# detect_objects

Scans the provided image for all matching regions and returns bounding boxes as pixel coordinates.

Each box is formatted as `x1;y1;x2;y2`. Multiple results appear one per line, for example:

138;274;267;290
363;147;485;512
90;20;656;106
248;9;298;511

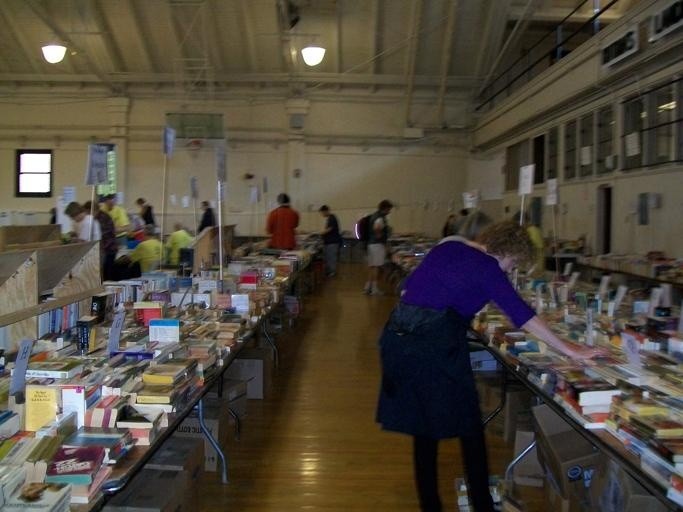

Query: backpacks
353;214;372;245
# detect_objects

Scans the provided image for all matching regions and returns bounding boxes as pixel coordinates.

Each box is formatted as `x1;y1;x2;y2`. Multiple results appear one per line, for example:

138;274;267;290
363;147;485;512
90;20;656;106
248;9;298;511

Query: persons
373;219;613;512
264;193;300;251
361;199;393;296
0;228;321;512
443;214;454;235
49;186;217;273
393;229;683;512
312;205;340;278
456;209;468;235
513;211;545;278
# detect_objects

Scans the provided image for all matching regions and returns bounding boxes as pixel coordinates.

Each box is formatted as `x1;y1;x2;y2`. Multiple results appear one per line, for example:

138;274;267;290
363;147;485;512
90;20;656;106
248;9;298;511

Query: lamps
301;36;328;67
41;33;68;64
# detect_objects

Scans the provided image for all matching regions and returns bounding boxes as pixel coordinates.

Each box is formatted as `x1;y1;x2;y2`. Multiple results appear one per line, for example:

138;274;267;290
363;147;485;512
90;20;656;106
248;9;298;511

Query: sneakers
363;287;385;297
